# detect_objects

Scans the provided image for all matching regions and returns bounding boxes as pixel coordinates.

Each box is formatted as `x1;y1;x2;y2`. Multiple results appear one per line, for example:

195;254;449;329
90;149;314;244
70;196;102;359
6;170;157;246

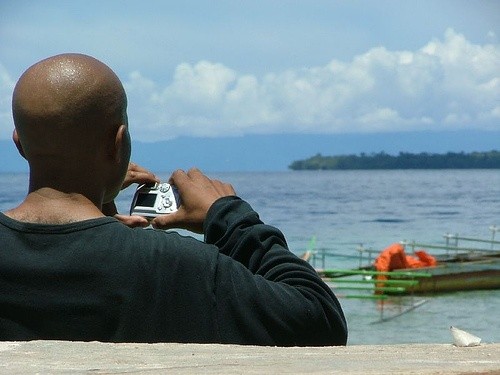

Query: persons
0;52;350;347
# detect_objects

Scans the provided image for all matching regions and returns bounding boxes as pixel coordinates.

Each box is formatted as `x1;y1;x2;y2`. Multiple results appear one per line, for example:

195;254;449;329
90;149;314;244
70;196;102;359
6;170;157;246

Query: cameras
130;182;183;225
